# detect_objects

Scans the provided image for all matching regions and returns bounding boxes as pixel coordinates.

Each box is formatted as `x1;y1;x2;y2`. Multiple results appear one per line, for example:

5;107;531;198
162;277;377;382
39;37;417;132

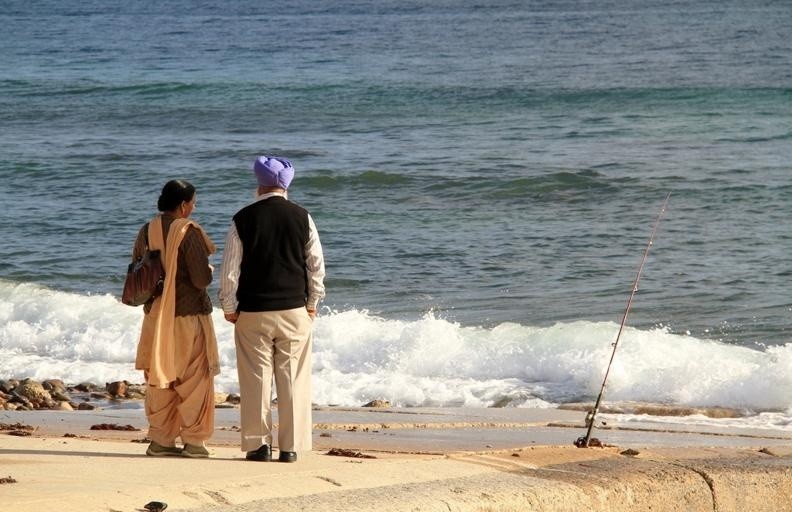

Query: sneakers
146;441;208;458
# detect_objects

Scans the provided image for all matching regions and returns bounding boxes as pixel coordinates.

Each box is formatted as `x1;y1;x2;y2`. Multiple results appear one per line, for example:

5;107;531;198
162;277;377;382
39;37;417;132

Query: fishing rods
577;191;670;447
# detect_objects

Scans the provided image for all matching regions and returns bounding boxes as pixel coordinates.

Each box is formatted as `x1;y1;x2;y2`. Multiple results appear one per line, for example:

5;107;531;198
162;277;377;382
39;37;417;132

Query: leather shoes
246;445;296;462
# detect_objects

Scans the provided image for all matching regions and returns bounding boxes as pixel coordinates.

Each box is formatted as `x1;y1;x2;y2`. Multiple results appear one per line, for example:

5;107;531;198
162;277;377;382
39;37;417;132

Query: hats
254;156;295;189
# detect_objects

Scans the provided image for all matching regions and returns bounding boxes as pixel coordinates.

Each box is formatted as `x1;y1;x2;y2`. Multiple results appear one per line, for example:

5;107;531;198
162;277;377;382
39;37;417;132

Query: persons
131;178;217;459
219;156;326;462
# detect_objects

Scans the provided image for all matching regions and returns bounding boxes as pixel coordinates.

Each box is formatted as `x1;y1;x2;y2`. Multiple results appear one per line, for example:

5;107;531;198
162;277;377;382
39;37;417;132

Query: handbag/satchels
122;250;165;306
